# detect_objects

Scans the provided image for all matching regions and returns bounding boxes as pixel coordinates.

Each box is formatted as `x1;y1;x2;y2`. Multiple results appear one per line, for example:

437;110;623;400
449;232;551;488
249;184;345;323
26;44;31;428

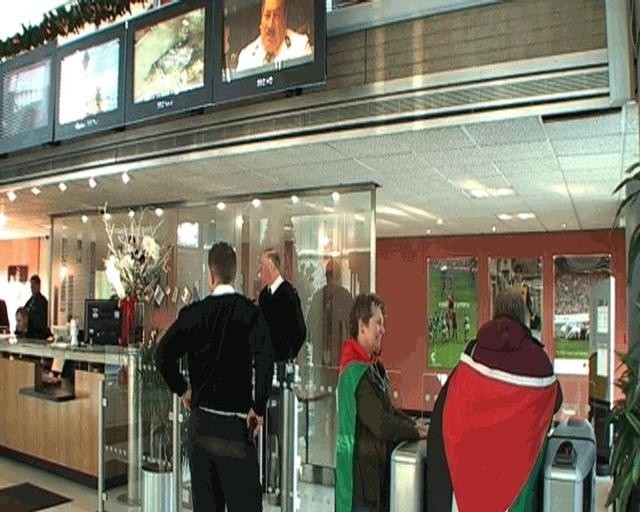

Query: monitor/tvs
85;297;118;345
212;0;328;106
54;21;124;143
0;43;55;158
126;0;211;126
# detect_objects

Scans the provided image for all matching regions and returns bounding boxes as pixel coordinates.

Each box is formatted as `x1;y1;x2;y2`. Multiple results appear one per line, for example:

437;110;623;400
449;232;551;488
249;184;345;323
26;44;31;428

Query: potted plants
126;323;189;512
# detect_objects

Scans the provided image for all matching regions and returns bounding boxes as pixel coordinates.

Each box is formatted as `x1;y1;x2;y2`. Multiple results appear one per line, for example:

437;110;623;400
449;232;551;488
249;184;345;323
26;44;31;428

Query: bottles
70;317;79;345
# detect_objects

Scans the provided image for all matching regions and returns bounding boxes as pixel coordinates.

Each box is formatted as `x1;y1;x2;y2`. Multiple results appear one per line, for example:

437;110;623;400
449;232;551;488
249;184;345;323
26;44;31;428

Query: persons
427;259;476;344
257;247;307;360
496;257;536;328
426;286;562;512
307;262;354;461
335;292;425;512
555;272;609;341
24;276;47;338
237;1;311;71
156;242;273;511
16;308;37;338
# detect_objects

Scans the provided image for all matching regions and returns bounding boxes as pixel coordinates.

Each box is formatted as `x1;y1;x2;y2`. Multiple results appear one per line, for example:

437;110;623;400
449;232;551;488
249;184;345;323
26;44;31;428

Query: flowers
97;201;172;303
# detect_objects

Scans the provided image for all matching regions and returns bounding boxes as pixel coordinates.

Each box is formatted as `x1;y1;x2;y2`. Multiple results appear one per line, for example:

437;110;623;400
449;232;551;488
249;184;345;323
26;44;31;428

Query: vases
120;297;137;348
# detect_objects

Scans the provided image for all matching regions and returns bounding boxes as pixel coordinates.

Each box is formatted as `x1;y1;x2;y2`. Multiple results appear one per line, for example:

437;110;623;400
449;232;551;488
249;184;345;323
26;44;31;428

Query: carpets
0;482;72;512
